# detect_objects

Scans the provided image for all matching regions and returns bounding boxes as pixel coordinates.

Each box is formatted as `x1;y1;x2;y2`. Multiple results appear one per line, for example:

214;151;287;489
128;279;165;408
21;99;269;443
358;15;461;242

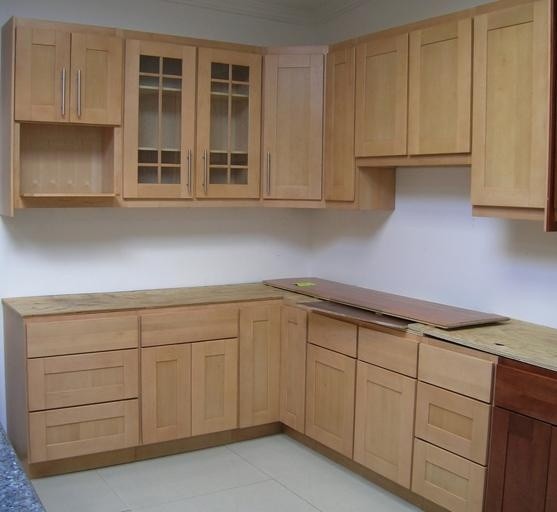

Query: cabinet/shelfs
239;298;282;440
411;335;499;512
3;304;140;479
262;45;325;210
0;17;124;219
483;355;556;511
324;40;395;212
473;1;557;231
124;29;261;208
355;9;472;167
282;304;307;445
308;308;419;507
140;302;239;463
